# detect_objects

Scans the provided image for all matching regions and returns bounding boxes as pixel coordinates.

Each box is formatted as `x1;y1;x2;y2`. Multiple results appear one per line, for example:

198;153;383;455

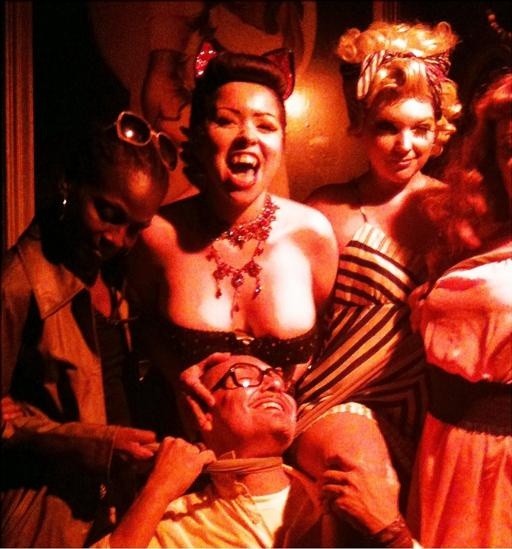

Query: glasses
209;362;289;390
103;111;177;172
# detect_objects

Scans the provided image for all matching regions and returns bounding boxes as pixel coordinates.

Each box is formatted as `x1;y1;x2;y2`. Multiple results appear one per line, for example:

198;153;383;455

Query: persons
1;23;511;547
